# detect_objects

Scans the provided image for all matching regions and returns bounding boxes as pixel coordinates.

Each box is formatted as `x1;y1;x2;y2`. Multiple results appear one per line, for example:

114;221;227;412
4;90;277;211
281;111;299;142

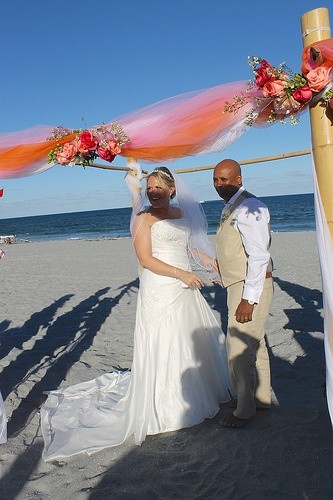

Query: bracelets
174;267;177;279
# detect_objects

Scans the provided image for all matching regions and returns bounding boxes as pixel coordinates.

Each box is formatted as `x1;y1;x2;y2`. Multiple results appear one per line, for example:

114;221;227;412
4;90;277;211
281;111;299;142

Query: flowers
222;55;333;127
46;123;131;168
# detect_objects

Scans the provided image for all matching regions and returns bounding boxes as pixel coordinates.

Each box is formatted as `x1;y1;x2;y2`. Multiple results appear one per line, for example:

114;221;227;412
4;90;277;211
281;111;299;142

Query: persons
214;159;274;426
129;167;237;451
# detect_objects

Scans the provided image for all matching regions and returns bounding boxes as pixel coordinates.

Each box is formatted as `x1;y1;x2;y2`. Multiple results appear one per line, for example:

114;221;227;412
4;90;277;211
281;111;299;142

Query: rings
245;317;248;319
